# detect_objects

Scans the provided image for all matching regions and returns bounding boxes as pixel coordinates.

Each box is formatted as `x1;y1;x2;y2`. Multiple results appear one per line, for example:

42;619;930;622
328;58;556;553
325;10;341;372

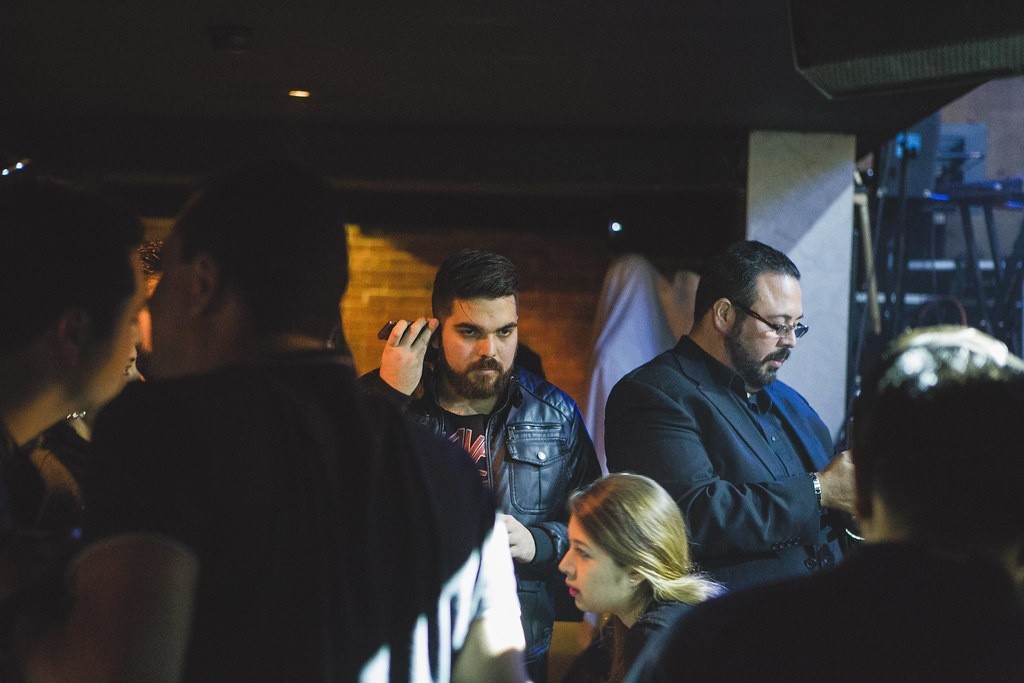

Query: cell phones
378;321;441;343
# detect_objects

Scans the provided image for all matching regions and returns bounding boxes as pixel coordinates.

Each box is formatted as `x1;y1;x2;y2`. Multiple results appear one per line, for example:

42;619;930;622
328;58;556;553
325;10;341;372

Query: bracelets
810;471;825;511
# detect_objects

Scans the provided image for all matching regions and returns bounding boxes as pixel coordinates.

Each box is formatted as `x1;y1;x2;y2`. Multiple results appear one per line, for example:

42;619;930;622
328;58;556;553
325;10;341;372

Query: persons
3;178;1023;683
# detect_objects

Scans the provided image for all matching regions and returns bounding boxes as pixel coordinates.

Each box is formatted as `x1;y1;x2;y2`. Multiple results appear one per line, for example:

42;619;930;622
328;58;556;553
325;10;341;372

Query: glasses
737;307;809;339
